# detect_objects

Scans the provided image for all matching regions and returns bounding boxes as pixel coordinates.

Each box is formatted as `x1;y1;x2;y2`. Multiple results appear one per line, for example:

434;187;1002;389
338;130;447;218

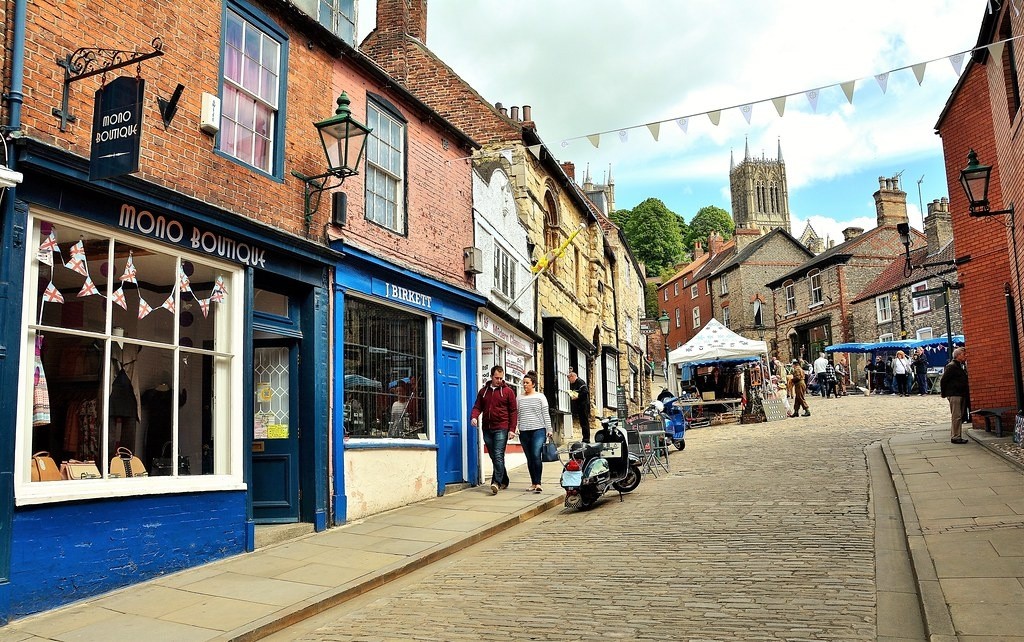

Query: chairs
622;412;670;479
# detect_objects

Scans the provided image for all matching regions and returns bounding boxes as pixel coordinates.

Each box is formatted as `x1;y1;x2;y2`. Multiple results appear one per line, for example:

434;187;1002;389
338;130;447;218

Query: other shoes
535;485;543;493
891;393;898;396
501;484;506;489
790;413;799;417
490;482;499;493
880;392;884;394
801;412;811;416
871;391;876;394
526;486;535;491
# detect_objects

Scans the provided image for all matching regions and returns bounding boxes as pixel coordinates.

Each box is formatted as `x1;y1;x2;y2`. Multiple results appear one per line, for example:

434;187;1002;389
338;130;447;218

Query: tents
669;318;774;399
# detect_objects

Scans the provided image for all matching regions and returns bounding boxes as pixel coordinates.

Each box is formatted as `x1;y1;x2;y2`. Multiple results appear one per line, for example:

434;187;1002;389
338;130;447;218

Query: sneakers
951;437;968;444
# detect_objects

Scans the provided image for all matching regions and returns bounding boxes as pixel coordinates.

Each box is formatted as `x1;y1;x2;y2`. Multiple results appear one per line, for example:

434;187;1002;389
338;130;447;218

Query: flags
530;228;582;272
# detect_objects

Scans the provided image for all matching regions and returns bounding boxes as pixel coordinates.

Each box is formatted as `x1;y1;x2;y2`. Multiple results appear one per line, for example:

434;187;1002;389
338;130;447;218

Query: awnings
825;335;965;367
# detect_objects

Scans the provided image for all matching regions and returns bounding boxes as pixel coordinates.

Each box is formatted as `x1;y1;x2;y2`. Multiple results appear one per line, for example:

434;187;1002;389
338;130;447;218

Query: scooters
553;415;643;512
642;388;687;456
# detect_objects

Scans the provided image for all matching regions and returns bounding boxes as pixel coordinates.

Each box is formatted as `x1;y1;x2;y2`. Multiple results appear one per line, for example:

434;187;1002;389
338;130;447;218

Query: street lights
658;308;670;387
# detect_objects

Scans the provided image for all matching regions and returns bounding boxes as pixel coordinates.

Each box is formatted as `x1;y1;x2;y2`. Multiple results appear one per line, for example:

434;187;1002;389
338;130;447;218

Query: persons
142;384;187;470
804;353;849;398
865;346;928;396
392;375;415;420
516;370;553;492
770;357;777;375
788;360;811;418
709;368;725;397
661;358;667;381
471;365;517;495
94;327;142;468
650;357;654;381
940;348;969;444
569;372;590;444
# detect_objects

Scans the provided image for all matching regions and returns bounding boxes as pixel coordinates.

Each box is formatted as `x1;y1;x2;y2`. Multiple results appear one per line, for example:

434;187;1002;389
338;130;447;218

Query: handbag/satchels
58;459;101;480
150;441;191;476
31;451;62;482
905;371;910;377
541;435;560;462
110;447;148;478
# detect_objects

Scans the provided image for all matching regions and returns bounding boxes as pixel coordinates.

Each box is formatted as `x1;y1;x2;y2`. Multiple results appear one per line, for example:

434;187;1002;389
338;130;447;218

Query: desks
639;430;669;476
680;398;743;429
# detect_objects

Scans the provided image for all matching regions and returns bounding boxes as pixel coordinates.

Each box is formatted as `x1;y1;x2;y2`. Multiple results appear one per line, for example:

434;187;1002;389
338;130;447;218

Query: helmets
650;400;664;412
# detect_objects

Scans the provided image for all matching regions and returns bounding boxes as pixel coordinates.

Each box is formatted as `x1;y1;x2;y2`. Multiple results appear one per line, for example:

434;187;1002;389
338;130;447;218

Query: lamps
290;90;373;226
958;147;1015;227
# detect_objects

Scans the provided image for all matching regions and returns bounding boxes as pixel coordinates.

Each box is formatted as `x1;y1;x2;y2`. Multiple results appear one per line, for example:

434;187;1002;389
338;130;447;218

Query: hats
791;359;799;363
